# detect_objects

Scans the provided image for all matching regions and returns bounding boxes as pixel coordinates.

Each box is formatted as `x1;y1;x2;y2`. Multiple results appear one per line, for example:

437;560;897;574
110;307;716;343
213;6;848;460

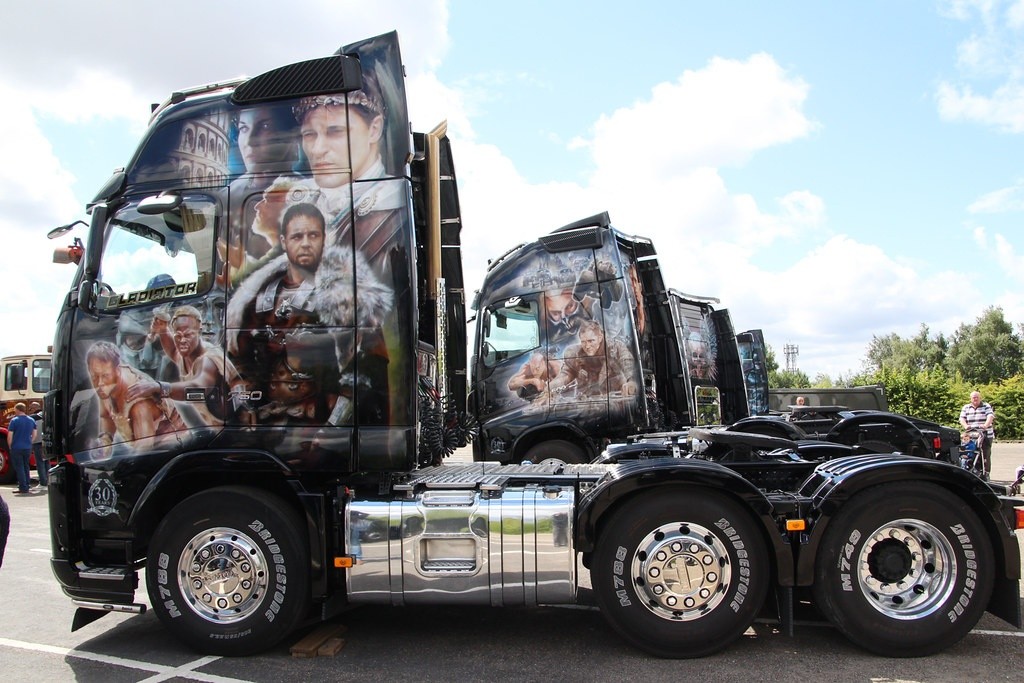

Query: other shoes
12;490;21;493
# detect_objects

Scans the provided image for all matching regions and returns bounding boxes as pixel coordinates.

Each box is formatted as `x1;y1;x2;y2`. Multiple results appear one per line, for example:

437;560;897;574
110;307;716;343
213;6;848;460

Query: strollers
958;427;990;482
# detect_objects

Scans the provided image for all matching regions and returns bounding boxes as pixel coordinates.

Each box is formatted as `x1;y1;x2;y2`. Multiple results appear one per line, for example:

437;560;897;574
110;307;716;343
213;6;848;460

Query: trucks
0;345;53;487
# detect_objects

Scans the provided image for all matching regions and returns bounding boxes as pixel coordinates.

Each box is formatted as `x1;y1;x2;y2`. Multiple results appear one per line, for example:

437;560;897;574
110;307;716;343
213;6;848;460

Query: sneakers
33;483;48;491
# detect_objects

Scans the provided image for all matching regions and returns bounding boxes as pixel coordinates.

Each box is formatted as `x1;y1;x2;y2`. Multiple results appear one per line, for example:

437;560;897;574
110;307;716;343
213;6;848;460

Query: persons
958;391;995;473
796;397;805;406
7;403;37;494
87;70;410;462
29;402;51;490
509;253;654;417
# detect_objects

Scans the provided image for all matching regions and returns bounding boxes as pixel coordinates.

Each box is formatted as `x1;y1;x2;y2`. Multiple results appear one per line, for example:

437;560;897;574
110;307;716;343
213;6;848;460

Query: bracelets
99;432;113;442
158;381;169;398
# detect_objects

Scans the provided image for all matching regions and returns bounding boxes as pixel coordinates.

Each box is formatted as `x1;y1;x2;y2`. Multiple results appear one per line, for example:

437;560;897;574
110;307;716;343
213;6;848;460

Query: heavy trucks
31;28;1024;661
464;208;963;466
